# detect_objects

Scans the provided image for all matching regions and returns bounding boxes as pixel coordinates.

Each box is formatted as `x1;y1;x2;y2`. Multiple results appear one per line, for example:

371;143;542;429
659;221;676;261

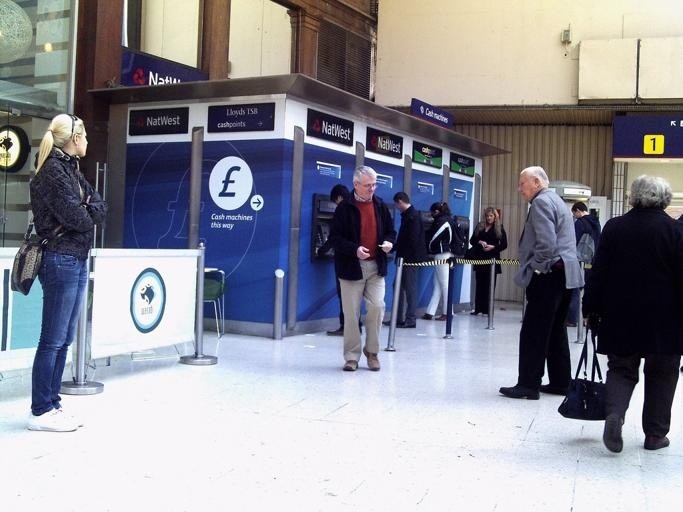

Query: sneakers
363;347;381;372
344;359;358;370
26;407;86;432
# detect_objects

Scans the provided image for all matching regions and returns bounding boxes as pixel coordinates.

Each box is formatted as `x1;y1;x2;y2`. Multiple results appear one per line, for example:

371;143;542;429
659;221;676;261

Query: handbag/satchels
465;246;476;260
557;378;609;422
11;241;43;295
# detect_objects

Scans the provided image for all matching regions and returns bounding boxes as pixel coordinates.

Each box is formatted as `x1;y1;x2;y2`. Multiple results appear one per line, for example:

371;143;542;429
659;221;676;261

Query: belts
361;255;376;262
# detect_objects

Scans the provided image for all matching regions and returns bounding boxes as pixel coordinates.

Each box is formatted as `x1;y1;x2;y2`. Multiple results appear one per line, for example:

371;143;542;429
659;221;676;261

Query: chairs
196;267;226;339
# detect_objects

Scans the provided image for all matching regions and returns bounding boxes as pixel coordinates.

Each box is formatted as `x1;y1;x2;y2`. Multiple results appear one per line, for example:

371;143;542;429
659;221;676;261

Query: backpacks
574;233;594;267
449;219;468;256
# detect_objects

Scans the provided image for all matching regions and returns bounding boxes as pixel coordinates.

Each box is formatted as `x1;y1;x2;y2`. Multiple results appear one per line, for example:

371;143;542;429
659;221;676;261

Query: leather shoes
435;314;453;321
397;318;417;328
541;380;570;395
603;411;624;453
383;319;391;326
326;326;346;336
499;383;541;402
643;434;670;450
421;313;432;320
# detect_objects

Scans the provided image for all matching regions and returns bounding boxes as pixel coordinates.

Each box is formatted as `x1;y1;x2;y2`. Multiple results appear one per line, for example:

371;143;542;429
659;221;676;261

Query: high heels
470;309;484;316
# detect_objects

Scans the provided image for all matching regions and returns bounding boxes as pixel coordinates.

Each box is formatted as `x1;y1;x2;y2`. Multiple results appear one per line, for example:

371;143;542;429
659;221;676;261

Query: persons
565;201;602;327
582;174;683;453
25;113;109;432
498;166;586;400
333;165;396;371
419;201;456;321
314;184;363;337
382;191;427;329
469;206;508;317
